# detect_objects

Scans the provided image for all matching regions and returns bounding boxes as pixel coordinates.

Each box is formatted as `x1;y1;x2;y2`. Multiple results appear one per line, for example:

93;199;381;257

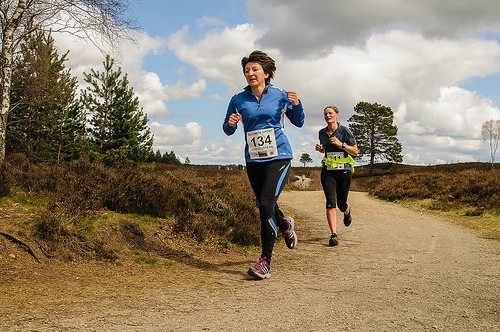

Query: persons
223;50;305;279
316;106;358;246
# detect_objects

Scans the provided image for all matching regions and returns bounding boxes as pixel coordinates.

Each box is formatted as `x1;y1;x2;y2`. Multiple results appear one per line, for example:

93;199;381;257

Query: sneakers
247;257;272;280
280;217;297;248
343;212;352;227
329;234;338;245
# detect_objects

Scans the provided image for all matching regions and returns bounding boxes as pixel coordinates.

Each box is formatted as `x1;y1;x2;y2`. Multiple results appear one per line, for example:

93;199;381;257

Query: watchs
339;142;346;149
